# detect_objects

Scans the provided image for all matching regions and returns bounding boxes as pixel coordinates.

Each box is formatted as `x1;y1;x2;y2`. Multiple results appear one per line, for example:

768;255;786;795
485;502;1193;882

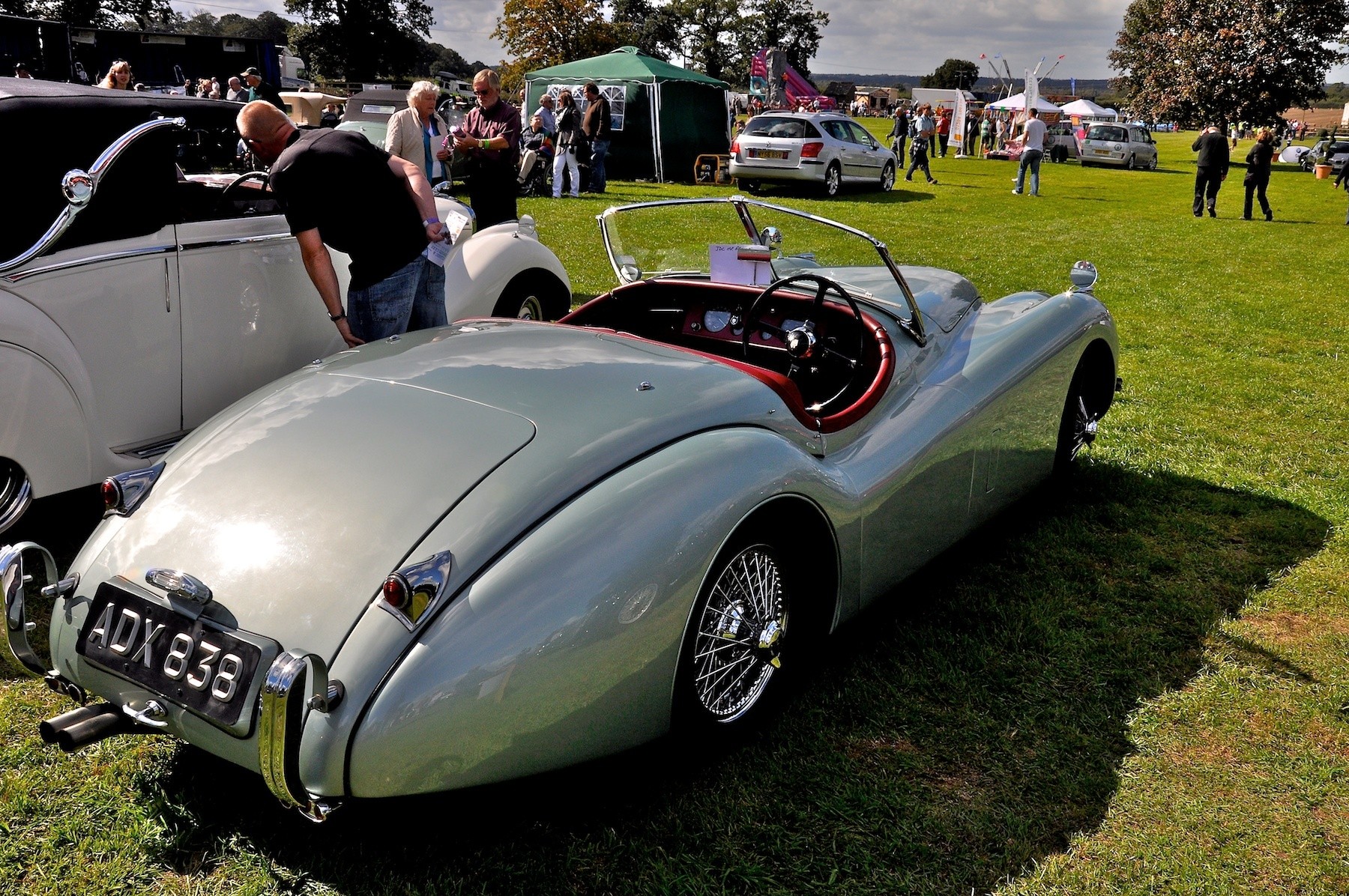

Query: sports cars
0;194;1123;832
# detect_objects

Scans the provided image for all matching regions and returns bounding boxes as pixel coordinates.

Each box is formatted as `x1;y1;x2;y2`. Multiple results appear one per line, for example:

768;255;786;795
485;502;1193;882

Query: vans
1043;120;1158;171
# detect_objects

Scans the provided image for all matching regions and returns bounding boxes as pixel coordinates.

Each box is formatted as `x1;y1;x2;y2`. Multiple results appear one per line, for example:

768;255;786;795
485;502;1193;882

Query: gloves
568;144;575;154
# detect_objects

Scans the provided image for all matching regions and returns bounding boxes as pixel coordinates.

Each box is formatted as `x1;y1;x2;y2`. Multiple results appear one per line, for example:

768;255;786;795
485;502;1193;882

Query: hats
1206;123;1220;129
239;67;261;77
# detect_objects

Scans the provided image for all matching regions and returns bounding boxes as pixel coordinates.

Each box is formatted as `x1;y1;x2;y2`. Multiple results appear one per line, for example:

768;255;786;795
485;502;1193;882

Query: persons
884;102;980;171
1238;130;1274;222
320;102;344;128
1172;119;1308;152
850;100;880;118
903;103;938;185
977;115;1007;158
746;95;821;117
91;59;135;91
520;82;612;198
236;100;451;350
382;80;457;191
454;67;522;234
135;67;288;116
1011;108;1049;196
1191;124;1230;218
1333;155;1349;226
12;61;36;79
728;93;746;135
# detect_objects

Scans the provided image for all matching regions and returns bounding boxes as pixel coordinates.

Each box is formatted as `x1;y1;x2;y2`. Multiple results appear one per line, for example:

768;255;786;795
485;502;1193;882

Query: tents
523;44;732;182
1058;98;1116;124
983;91;1064;145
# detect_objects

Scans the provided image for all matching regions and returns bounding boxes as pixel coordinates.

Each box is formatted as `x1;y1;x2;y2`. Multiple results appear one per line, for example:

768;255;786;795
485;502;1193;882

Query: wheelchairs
515;131;570;196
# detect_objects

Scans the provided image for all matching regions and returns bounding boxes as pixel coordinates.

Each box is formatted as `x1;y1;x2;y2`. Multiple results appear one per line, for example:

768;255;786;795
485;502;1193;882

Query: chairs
1041;147;1059;164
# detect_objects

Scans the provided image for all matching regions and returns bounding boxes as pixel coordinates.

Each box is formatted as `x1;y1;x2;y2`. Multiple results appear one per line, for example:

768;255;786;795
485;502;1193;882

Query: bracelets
484;138;489;149
327;306;345;322
423;217;439;227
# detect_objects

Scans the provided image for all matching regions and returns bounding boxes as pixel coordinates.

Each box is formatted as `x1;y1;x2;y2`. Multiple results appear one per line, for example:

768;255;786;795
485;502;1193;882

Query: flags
1041;56;1045;61
980;54;987;59
1059;55;1066;59
995;51;1002;58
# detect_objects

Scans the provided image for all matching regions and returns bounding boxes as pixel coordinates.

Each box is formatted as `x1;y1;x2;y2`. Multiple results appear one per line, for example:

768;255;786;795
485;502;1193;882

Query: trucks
910;87;979;111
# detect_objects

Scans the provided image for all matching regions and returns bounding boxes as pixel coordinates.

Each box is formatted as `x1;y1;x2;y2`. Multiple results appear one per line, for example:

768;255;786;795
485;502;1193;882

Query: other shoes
515;176;525;186
584;190;595;195
1207;206;1217;218
1195;214;1202;218
1239;216;1252;221
1264;208;1273;222
1027;193;1032;197
1011;189;1020;196
937;155;943;158
903;178;913;183
1011;178;1019;182
930;178;938;185
551;195;558;199
567;195;575;199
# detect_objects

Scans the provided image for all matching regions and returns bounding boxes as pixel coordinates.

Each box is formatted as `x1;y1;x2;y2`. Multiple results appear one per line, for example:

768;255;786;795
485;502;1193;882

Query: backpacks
908;118;918;137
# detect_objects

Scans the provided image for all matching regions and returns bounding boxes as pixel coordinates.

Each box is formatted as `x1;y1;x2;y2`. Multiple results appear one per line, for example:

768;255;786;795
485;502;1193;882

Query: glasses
472;87;490;96
547;100;554;103
558;99;562;103
245;137;262;157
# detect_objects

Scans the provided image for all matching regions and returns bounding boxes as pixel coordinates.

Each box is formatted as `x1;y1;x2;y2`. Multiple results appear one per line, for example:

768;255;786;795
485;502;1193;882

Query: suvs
728;109;898;197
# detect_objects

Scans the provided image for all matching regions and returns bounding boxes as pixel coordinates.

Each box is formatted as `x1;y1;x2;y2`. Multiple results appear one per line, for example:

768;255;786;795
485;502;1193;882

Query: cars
1300;141;1349;173
1;75;571;564
278;89;456;181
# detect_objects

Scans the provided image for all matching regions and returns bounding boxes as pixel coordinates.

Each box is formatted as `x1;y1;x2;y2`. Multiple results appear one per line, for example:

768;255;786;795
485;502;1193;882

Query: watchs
476;138;483;148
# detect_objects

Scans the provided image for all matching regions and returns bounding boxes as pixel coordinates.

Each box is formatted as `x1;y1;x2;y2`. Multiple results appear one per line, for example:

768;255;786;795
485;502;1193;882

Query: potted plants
1269;126;1284;162
1306;125;1337;178
